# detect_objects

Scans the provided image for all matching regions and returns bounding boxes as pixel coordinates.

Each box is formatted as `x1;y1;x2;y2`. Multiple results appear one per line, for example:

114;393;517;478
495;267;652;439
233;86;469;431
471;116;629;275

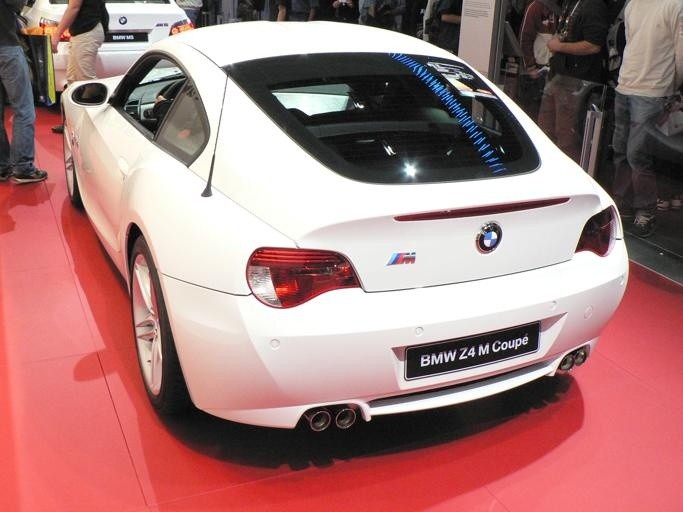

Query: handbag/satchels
18;33;56;107
533;32;555;65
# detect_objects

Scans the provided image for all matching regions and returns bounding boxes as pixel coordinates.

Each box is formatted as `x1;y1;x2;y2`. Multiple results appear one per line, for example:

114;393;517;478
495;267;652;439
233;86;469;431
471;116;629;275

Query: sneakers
0;169;14;180
656;196;682;211
620;211;659;237
15;169;47;183
51;125;64;132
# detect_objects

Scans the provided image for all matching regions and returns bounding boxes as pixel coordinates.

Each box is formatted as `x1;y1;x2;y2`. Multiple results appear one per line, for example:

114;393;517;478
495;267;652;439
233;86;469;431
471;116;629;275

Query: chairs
373;74;458;159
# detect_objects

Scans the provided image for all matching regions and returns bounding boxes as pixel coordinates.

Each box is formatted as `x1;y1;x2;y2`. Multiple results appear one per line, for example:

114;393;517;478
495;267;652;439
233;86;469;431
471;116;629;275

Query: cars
14;0;195;114
60;20;630;432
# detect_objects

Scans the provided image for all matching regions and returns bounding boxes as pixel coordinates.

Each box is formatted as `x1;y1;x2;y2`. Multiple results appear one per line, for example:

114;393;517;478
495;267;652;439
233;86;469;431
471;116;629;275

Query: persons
194;0;463;57
51;0;105;134
150;94;202;136
498;1;626;163
0;0;48;184
609;0;681;239
